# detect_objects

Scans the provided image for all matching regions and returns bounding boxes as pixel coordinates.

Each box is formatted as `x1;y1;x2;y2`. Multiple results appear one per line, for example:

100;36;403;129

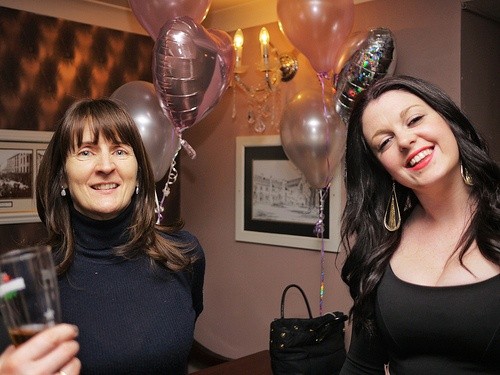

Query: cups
0;244;64;348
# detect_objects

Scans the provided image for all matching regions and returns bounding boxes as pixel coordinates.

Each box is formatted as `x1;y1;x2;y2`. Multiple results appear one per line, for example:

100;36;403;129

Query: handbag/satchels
270;284;349;375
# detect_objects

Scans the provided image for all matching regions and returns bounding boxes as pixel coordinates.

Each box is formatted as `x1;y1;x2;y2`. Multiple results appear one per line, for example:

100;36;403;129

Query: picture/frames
235;135;341;254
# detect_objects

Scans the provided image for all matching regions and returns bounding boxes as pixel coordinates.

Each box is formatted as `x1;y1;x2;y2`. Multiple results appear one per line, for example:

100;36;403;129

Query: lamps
229;27;297;134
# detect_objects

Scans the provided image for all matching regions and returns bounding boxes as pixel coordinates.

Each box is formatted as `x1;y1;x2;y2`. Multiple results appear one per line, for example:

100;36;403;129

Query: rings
58;370;69;375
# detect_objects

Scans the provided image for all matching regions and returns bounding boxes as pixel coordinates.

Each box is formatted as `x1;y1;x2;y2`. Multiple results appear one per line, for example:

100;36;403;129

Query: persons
0;99;206;375
339;75;500;375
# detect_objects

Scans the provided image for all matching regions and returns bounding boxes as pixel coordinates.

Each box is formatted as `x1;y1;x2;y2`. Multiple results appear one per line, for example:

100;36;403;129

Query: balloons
153;16;236;133
129;0;212;42
109;81;177;182
277;0;354;73
279;90;347;190
334;26;398;125
